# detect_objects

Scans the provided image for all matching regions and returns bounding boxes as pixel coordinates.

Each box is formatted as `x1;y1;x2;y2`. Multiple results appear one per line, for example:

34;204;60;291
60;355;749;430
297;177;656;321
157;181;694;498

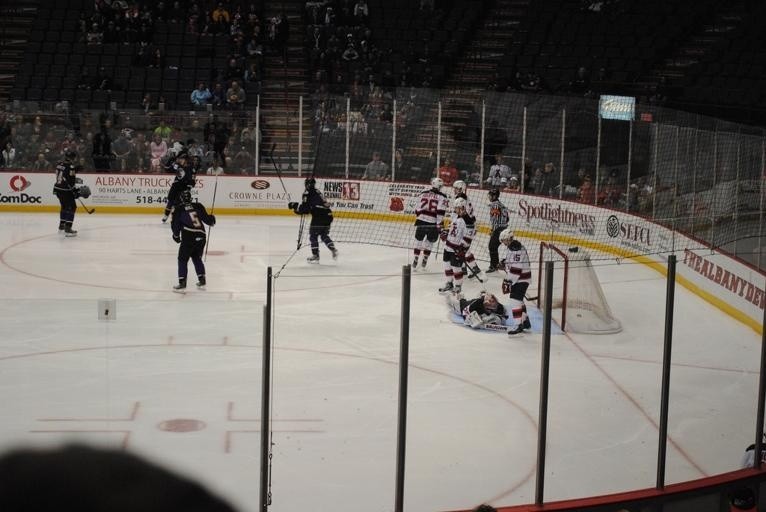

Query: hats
509;176;517;180
500;177;507;183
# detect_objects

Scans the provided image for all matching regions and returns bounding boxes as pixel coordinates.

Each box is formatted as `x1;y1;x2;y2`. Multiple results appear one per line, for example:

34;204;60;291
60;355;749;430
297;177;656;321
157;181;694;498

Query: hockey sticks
270;144;291;203
204;174;217;262
452;245;489;283
62;176;95;214
503;268;539;301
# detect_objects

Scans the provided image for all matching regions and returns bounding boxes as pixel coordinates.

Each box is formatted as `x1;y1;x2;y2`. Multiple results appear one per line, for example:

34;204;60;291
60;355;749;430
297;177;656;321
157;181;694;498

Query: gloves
436;224;442;231
288;202;298;209
172;234;180;242
501;279;511;294
440;230;449;241
73;192;80;198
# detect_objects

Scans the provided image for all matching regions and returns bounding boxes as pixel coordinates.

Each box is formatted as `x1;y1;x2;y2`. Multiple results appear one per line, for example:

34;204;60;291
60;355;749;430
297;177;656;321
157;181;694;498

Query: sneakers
59;225;65;229
64;226;77;233
307;255;318;260
412;256;418;267
523;317;530;329
468;268;480;278
485;267;496;273
422;256;428;267
173;281;186;289
162;215;167;222
439;282;453;292
196;277;206;286
332;248;337;257
508;325;524;334
452;285;461;292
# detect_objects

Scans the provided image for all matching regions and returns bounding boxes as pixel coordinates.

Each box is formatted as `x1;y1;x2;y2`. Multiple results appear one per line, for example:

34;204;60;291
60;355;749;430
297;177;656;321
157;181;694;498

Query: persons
411;177;450;272
440;154;481;188
416;152;437;183
52;152;89;236
161;151;196;222
440;180;483;280
393;149;412;182
498;228;532;337
521;158;708;221
171;191;216;295
1;0;299;173
299;1;659;139
440;291;509;328
361;152;392;184
481;153;521;193
485;188;510;274
295;177;339;264
438;197;476;302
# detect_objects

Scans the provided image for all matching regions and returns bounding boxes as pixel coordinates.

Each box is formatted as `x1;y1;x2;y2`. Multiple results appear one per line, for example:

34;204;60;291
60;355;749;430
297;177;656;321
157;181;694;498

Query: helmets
178;154;188;158
65;151;75;158
498;229;513;242
431;178;443;188
453;180;466;190
305;177;316;184
454;197;466;207
179;191;191;202
487;189;499;199
